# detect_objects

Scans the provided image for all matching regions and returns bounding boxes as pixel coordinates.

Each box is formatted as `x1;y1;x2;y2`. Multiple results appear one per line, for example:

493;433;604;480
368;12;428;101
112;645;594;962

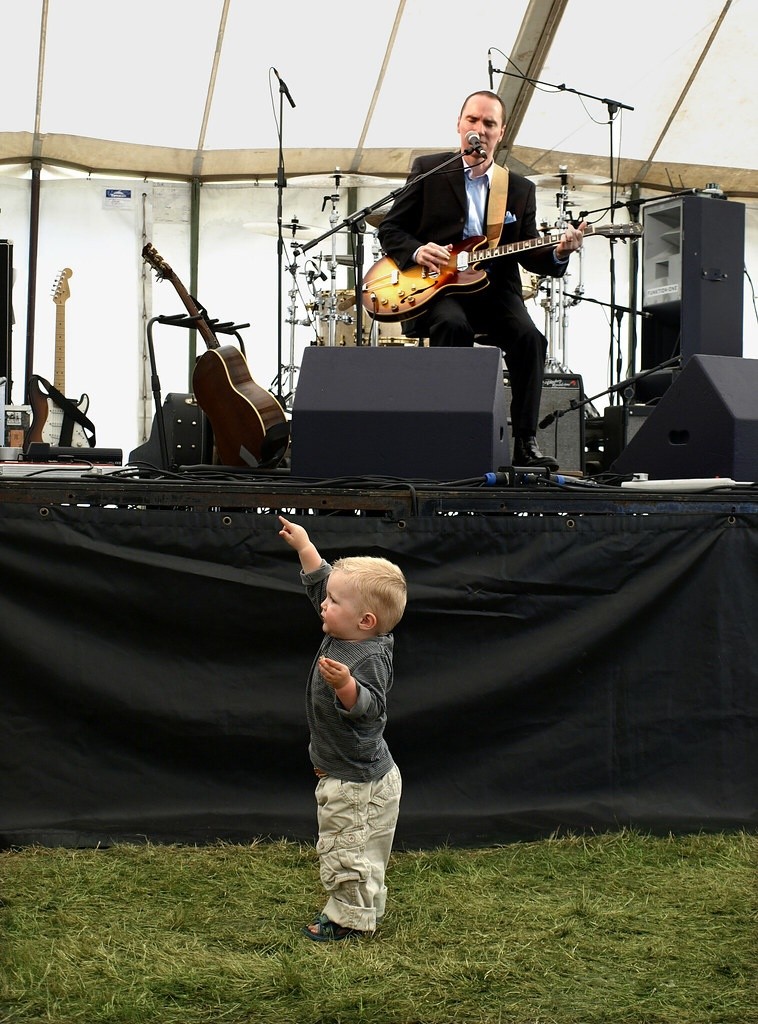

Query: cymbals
536;190;603;204
525;172;614;189
288;172;390;188
244;220;332;241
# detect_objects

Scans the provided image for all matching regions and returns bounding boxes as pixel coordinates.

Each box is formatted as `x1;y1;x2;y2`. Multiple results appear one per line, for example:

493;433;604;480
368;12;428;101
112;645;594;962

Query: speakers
607;354;758;487
642;198;745;371
500;371;586;480
604;406;656;467
291;345;512;482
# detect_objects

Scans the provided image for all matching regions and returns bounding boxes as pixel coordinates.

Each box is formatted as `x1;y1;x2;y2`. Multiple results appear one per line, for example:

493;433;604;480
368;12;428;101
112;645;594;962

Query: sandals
301;916;356;944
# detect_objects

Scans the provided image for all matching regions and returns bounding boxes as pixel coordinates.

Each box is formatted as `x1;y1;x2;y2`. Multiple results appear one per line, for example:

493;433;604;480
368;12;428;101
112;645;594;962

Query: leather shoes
512;433;559;473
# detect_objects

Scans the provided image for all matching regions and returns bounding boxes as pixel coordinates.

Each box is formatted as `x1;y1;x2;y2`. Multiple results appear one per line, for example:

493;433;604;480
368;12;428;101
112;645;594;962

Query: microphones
538;410;565;429
466;131;488;159
273;67;296;109
311;261;328;281
487;49;493;89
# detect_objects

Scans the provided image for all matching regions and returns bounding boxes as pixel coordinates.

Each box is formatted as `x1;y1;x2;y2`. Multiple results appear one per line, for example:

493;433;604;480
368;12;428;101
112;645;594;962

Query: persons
378;91;587;472
278;515;406;943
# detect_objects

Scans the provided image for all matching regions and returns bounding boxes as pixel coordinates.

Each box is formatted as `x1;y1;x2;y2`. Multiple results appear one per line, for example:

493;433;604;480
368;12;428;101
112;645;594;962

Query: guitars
352;222;647;324
141;244;288;469
42;268;89;448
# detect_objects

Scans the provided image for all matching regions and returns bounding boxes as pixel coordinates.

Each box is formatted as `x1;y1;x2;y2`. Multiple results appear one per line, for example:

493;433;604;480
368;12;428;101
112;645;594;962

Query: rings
566;240;572;242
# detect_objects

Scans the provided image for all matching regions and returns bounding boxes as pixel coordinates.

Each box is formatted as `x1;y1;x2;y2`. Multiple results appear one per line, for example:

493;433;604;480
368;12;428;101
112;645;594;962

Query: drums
315;289;369;346
361;336;420;347
518;262;538;299
